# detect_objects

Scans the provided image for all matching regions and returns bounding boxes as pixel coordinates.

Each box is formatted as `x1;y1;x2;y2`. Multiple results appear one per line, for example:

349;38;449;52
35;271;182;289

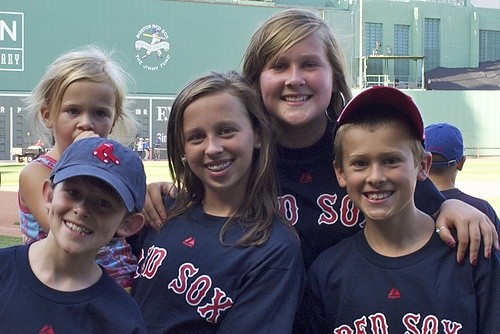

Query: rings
435;225;447;236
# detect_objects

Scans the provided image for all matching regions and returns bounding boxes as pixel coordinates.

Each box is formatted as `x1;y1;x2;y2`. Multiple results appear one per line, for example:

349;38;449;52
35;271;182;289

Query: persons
301;87;500;334
129;71;306;334
137;134;161;160
0;137;148;334
421;122;500;243
140;10;500;266
18;45;137;292
